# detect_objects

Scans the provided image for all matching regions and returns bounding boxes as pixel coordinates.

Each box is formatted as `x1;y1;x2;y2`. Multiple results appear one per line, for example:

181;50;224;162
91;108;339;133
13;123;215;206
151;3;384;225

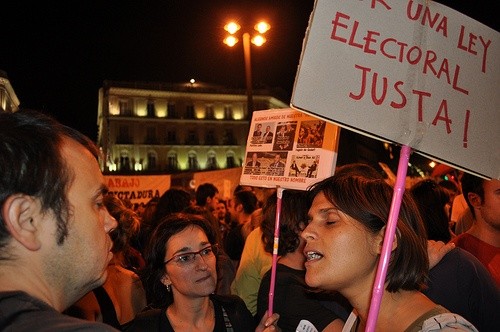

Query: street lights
220;17;272;123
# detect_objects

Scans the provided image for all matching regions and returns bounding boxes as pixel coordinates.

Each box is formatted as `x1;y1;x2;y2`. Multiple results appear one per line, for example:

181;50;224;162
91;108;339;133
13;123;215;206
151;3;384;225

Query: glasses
164;245;218;266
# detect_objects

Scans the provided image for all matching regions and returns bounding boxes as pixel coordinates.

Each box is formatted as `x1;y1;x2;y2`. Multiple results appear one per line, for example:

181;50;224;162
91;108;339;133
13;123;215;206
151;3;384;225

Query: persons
243;122;326;177
0;111;121;332
61;163;500;332
